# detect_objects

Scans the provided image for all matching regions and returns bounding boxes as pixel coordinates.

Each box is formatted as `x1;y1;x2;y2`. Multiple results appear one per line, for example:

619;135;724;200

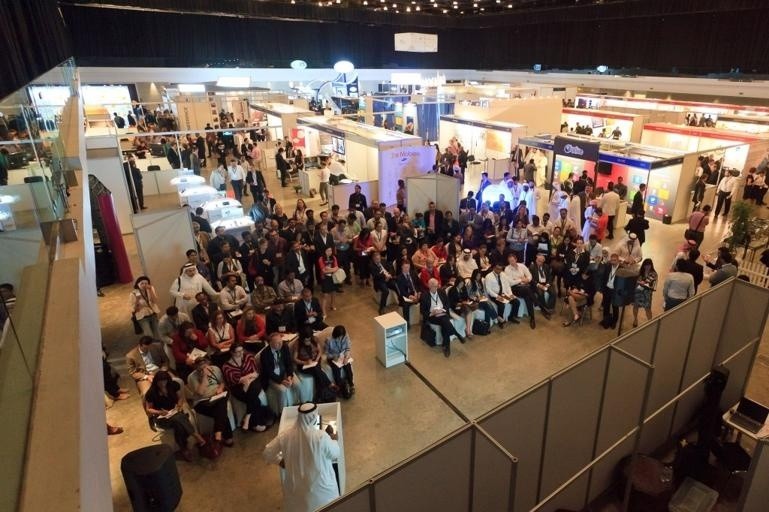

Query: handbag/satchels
332;267;347;284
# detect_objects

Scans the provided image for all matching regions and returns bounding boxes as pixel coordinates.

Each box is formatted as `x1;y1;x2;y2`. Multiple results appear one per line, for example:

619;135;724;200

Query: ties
377;262;384;272
497;274;502;296
275;350;282;366
405;274;414;294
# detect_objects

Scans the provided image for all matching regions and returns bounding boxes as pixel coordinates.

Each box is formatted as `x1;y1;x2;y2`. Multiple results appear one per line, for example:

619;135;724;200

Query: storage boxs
669;477;719;512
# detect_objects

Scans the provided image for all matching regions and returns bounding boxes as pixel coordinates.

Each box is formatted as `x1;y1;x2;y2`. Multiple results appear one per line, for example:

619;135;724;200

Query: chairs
155;314;353;452
0;291;33;382
371;260;603;347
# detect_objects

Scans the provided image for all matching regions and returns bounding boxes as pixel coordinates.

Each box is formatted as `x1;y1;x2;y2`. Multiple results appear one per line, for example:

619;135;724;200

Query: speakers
120;443;184;512
612;267;640;307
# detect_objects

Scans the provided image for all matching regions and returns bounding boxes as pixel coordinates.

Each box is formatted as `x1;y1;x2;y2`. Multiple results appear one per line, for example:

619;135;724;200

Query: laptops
729;395;769;434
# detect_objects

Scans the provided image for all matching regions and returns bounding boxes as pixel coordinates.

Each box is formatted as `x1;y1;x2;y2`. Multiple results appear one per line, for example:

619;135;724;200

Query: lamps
394;32;438;52
596;56;609;73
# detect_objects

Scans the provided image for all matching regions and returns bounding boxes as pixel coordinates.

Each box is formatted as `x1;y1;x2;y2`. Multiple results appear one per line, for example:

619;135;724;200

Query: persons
99;96;768;461
0;284;16;311
264;402;340;512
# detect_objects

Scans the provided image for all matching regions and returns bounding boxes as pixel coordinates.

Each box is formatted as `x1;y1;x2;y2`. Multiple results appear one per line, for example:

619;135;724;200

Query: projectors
386;326;402;338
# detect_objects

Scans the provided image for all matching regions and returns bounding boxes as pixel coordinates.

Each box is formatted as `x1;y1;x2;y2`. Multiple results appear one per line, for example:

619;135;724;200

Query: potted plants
728;200;754;241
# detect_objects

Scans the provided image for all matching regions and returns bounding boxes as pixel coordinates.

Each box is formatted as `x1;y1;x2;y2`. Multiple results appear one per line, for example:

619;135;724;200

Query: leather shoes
177;385;352;462
108;426;124;434
442;305;638;358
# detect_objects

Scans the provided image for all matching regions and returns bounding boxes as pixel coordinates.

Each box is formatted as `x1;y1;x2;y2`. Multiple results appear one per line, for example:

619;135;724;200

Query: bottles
660;465;674;483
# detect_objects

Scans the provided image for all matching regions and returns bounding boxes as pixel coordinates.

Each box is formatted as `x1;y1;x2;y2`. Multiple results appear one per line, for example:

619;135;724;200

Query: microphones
319;415;322;429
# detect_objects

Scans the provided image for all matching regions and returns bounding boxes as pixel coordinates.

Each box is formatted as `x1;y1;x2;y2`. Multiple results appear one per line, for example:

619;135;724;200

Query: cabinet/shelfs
374;311;408;368
737;436;769;511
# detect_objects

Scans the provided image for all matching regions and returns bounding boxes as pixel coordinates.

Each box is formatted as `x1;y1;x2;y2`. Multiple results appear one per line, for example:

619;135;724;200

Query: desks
724;233;769;263
596;195;628;229
171;174;255;238
687;184;717;220
234;132;378;210
